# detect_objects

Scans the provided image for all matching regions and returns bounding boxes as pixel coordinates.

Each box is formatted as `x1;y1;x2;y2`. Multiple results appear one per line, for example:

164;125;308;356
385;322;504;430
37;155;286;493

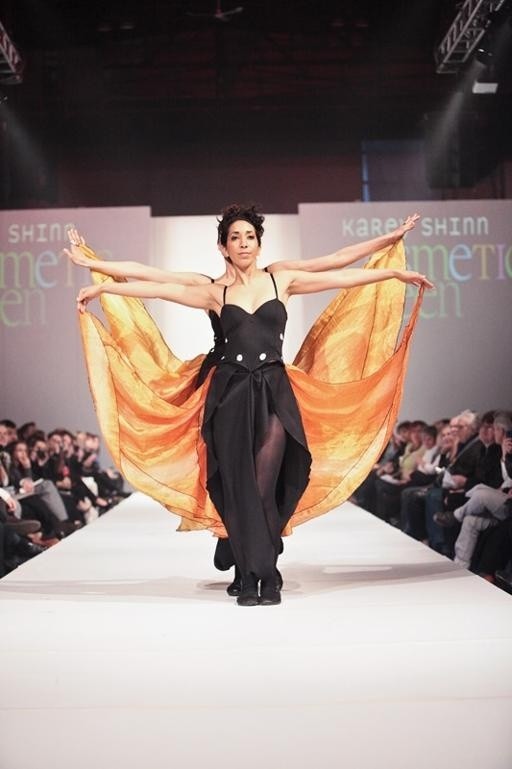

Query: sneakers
4;515;42;535
433;512;461;530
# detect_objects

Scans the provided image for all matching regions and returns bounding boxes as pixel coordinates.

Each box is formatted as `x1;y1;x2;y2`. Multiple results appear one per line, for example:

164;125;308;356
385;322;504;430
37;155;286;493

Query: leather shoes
55;520;84;540
227;567;283;605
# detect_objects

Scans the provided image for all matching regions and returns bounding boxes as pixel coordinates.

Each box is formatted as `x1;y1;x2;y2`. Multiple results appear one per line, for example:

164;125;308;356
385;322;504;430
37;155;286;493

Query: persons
0;419;132;579
349;408;511;570
